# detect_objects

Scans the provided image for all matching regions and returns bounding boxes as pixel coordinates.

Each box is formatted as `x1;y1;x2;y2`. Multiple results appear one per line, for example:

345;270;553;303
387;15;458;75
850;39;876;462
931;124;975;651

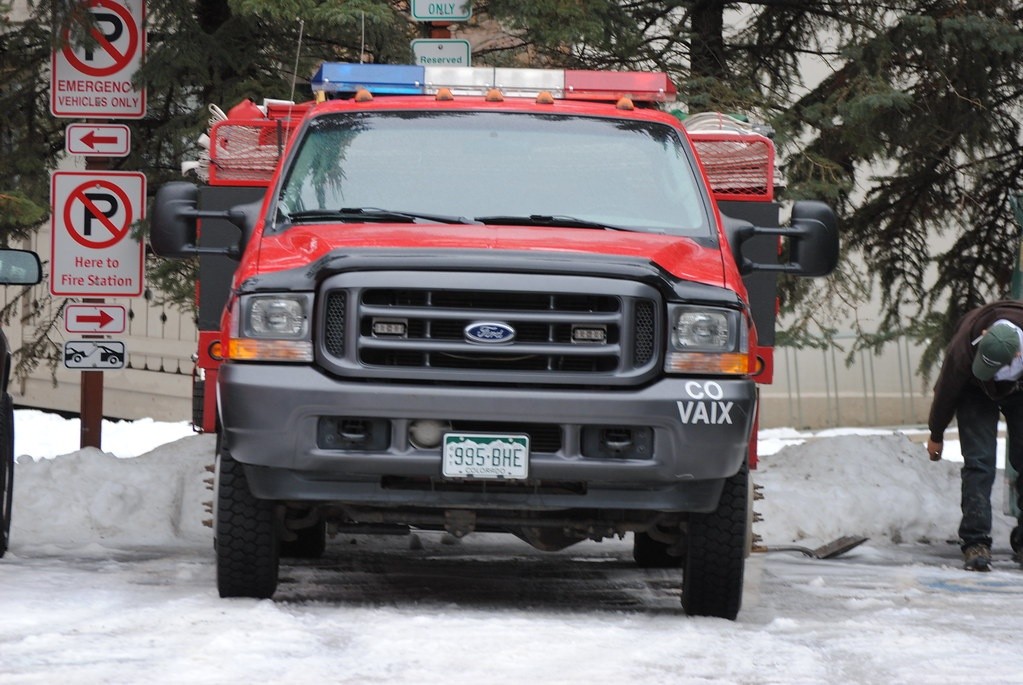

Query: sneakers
964;543;991;571
1011;549;1023;564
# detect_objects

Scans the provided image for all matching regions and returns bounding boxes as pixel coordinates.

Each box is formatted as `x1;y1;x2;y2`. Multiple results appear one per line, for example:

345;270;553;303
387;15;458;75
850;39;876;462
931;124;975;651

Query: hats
972;323;1019;381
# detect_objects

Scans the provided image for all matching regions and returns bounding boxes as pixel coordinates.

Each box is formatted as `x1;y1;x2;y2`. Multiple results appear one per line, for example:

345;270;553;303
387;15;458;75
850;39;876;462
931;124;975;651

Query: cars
0;248;42;563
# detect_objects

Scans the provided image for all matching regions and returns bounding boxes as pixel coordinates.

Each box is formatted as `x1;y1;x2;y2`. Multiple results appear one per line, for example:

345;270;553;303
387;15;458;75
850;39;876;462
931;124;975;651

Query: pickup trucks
147;58;840;621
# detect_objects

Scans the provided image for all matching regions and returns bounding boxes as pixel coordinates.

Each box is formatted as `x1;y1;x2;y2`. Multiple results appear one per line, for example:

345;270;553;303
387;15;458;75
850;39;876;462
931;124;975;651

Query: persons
927;300;1023;573
